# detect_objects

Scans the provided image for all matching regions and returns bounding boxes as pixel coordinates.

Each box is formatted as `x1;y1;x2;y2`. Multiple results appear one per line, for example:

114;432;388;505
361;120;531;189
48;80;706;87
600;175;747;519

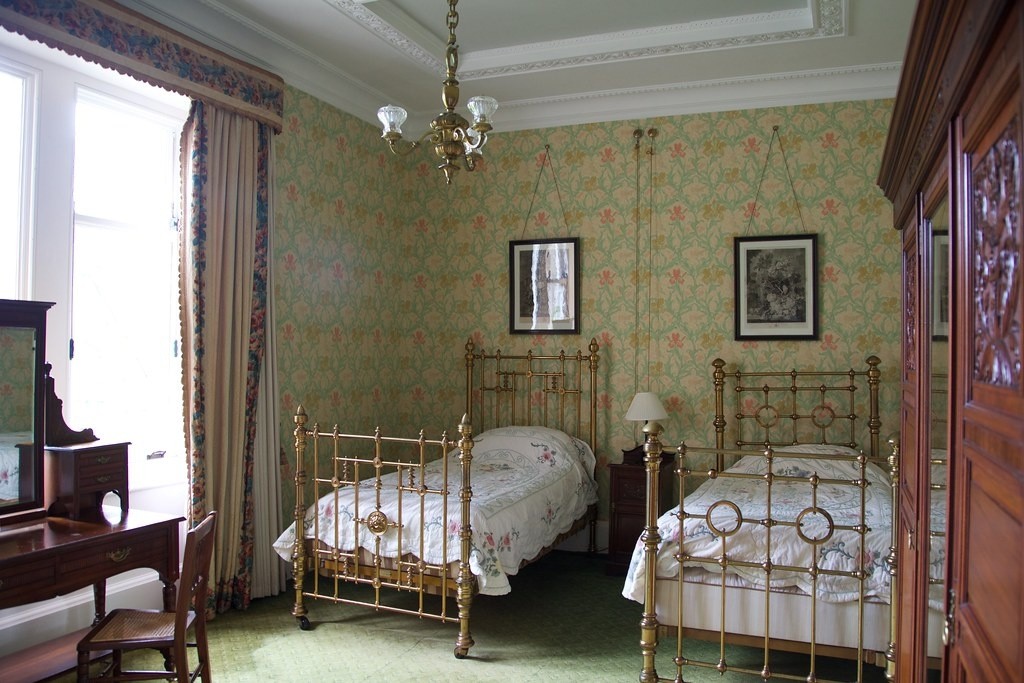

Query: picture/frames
508;236;581;335
733;233;821;342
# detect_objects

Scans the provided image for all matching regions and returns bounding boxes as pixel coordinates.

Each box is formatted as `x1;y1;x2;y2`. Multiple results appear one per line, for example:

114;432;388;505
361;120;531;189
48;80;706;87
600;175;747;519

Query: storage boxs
44;439;132;522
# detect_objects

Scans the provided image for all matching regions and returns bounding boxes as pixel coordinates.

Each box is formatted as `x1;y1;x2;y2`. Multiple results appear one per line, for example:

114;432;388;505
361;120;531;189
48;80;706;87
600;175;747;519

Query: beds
638;349;901;683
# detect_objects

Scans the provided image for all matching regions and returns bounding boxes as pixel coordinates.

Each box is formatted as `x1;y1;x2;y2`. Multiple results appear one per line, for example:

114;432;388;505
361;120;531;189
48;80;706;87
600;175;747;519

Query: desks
0;503;188;683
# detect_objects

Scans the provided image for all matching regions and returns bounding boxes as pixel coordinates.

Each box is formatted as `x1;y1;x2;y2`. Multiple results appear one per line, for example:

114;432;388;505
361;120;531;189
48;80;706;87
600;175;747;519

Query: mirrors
924;189;952;683
0;298;57;531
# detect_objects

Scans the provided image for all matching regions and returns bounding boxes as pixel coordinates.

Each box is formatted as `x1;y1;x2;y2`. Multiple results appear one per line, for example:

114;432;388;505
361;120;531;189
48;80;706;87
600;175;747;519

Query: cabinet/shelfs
878;0;1024;683
606;444;676;577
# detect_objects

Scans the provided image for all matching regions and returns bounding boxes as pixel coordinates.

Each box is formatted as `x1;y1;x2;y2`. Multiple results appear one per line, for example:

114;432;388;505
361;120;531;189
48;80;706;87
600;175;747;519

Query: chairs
75;510;218;683
271;337;604;660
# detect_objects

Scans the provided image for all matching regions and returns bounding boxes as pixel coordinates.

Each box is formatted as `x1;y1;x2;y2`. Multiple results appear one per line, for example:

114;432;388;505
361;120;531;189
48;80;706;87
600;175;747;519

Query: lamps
377;0;499;185
623;392;669;466
